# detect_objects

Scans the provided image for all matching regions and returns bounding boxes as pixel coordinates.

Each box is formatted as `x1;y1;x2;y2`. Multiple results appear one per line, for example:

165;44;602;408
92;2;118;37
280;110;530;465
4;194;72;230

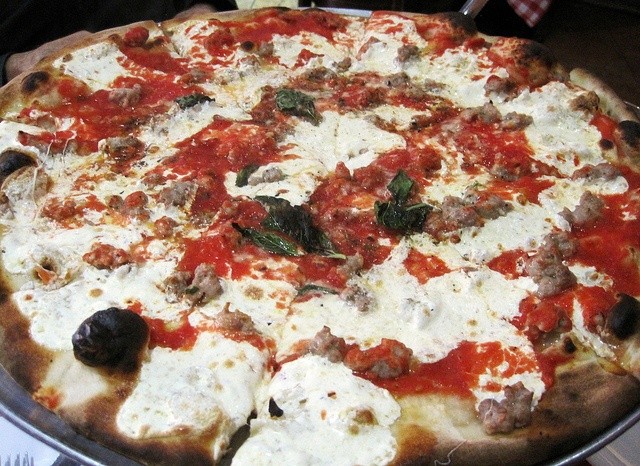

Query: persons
0;1;237;86
